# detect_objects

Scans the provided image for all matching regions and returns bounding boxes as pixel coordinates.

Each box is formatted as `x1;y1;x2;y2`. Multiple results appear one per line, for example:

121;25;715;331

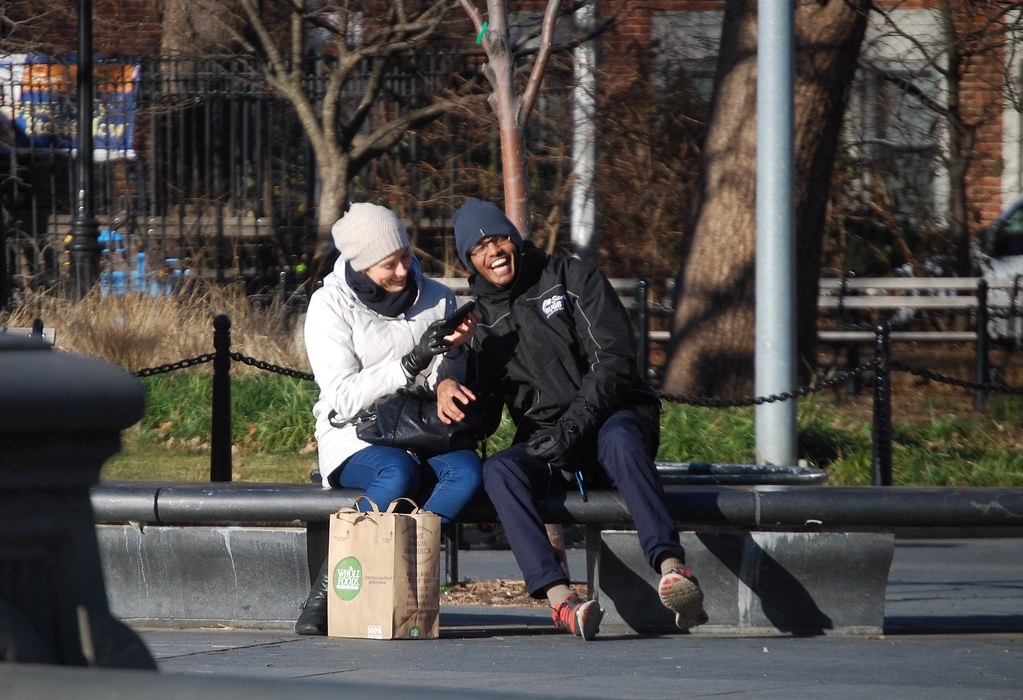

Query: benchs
429;275;1023;412
47;213;273;277
311;465;827;629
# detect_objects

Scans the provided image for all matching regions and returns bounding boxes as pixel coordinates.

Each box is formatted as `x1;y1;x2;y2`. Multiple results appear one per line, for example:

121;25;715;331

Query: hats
332;203;414;272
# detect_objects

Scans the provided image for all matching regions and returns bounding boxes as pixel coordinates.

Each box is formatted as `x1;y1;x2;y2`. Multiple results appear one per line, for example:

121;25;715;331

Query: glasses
469;234;511;256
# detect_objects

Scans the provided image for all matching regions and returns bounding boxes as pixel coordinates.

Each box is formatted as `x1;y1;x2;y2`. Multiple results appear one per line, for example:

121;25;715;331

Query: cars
962;192;1023;348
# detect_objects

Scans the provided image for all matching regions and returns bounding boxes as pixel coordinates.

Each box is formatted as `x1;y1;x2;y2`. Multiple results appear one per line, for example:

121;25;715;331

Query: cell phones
442;301;475;331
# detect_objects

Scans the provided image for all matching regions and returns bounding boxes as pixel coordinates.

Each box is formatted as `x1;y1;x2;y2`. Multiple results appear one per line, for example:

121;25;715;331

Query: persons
423;197;710;641
294;201;487;637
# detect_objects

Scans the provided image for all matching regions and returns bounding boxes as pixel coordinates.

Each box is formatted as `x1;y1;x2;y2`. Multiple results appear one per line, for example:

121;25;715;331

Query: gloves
401;319;454;377
527;419;580;467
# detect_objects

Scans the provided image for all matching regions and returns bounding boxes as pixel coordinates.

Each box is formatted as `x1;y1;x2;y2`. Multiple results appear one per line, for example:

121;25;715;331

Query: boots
295;558;328;636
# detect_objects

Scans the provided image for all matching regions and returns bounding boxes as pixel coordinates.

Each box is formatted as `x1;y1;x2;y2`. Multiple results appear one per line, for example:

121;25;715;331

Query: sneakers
659;567;708;629
549;592;606;640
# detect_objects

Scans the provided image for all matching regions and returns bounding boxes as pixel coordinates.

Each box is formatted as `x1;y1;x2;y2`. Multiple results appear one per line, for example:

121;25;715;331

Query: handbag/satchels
328;495;441;640
353;388;482;458
